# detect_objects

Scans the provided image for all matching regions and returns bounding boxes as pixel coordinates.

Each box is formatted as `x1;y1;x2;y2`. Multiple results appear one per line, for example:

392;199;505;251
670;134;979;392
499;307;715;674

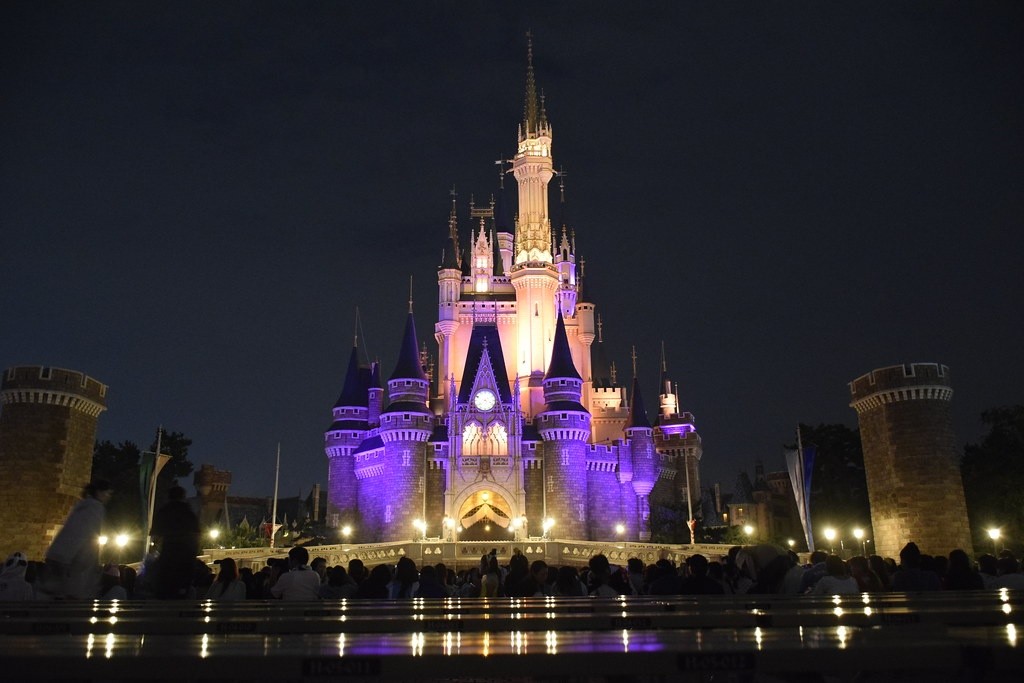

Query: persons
0;521;1024;603
45;479;112;573
680;555;724;595
150;503;201;600
268;546;320;603
896;542;941;590
814;554;858;595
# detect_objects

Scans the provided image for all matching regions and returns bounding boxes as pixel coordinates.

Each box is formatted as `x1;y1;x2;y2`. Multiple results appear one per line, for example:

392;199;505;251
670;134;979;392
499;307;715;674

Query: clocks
475;391;495;411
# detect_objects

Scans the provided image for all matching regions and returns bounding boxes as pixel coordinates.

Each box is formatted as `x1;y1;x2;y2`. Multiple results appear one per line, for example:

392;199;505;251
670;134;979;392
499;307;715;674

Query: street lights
547;519;555;541
990;529;1000;557
855;530;866;553
210;530;218;548
99;536;106;560
446;519;454;543
745;526;753;544
412;520;420;542
825;529;834;551
115;534;128;564
